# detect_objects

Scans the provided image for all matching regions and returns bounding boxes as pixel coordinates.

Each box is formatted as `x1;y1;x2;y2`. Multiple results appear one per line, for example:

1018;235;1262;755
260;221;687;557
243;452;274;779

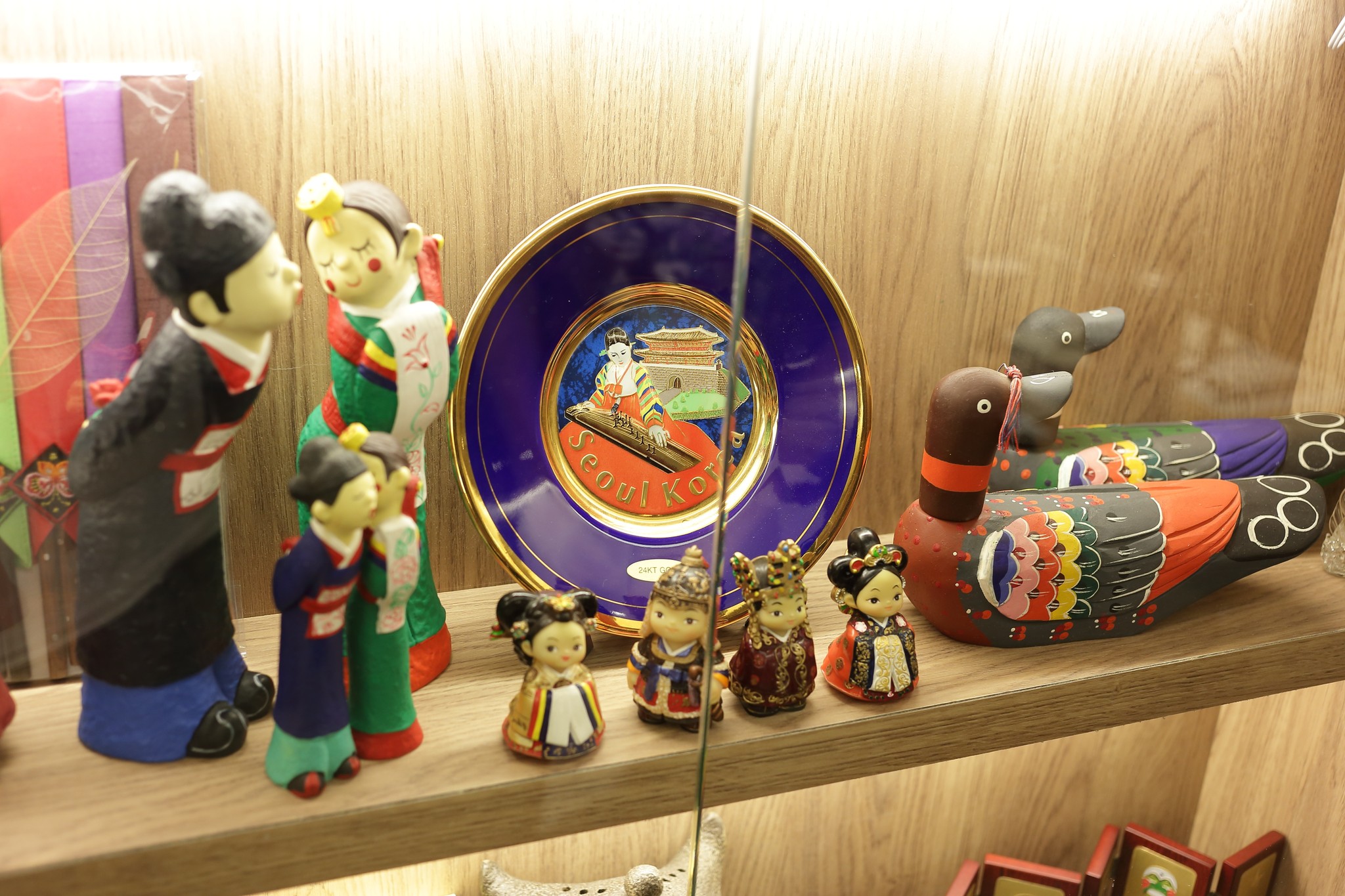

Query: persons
820;526;921;704
264;423;429;799
729;537;818;716
73;167;307;767
287;173;458;696
625;544;729;734
494;586;608;762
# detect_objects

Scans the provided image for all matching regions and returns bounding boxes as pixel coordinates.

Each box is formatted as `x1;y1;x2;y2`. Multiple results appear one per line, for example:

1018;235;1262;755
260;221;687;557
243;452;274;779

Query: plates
446;184;872;639
